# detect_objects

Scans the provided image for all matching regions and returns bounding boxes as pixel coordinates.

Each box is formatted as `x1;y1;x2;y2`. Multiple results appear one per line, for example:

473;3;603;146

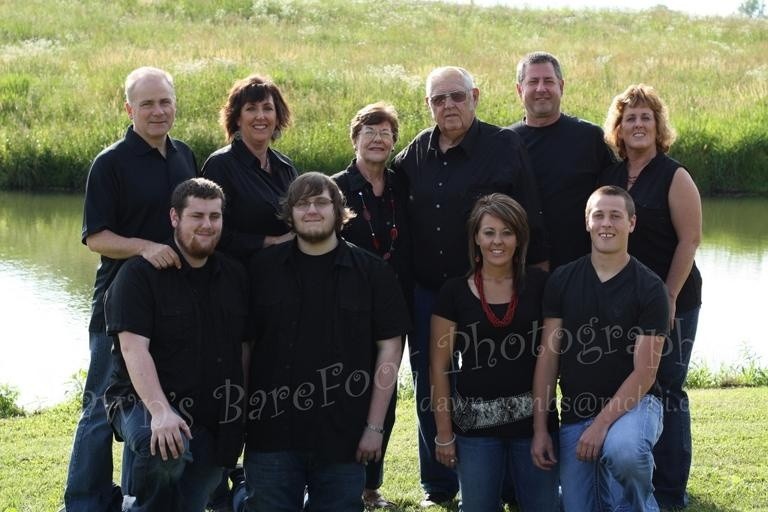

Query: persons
601;81;704;511
529;184;672;511
391;65;550;508
103;177;247;511
229;172;414;512
327;98;412;511
501;51;621;273
64;65;202;511
202;72;301;511
428;191;559;511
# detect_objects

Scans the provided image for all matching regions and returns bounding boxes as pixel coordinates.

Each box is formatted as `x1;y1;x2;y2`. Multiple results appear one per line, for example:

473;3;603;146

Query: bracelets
364;419;386;435
434;431;457;446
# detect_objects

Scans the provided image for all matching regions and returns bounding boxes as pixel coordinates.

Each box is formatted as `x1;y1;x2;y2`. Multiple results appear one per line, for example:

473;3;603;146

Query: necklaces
625;174;640;183
472;263;526;327
349;169;400;263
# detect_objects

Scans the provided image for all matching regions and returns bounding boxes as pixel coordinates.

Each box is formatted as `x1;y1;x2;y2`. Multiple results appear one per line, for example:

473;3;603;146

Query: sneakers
363;493;395;511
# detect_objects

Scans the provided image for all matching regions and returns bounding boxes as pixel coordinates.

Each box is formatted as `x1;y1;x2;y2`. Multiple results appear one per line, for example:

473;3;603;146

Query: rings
449;458;457;465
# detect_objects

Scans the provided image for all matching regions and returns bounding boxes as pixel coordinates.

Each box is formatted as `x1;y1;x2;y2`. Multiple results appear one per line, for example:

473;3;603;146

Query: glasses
292;196;331;210
430;90;468;107
363;127;394;138
